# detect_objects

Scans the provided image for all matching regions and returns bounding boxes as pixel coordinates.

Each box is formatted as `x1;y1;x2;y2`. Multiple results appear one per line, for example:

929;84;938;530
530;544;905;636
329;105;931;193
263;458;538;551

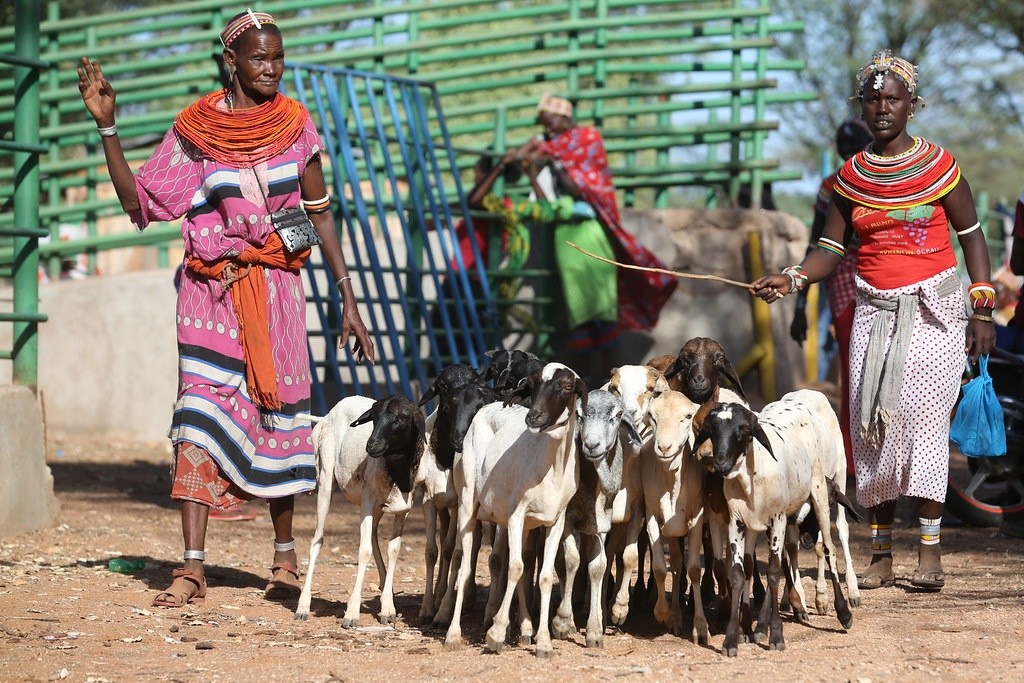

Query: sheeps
293;391;429;628
416;335;862;660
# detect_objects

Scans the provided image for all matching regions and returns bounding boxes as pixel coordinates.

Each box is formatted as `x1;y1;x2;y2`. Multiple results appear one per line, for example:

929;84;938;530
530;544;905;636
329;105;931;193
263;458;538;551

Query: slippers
911;569;945;586
856;573;895;588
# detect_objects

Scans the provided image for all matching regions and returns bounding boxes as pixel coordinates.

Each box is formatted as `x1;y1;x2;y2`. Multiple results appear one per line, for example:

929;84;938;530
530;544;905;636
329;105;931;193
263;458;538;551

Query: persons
432;146;516;356
789;116;874;476
76;11;374;607
518;97;677;348
748;50;997;587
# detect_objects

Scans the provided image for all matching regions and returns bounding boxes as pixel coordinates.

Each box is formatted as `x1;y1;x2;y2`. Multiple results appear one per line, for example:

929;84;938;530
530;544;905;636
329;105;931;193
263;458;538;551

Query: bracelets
782;266;808;293
968;282;996;310
336;277;351;286
972;314;993;322
96;124;117;136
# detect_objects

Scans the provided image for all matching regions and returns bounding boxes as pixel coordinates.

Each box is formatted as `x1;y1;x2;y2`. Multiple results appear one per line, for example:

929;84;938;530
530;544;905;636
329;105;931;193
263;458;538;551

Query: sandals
264;563;301;601
152;568;207;606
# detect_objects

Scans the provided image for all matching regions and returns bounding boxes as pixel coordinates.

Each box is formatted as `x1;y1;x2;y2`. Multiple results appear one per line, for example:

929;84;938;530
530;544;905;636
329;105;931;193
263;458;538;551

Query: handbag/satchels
949;352;1007;458
271;206;323;253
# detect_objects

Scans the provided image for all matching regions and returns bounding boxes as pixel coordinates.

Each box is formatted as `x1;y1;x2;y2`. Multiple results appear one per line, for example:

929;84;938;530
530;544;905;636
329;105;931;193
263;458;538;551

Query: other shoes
567;327;595;354
594;320;622;348
